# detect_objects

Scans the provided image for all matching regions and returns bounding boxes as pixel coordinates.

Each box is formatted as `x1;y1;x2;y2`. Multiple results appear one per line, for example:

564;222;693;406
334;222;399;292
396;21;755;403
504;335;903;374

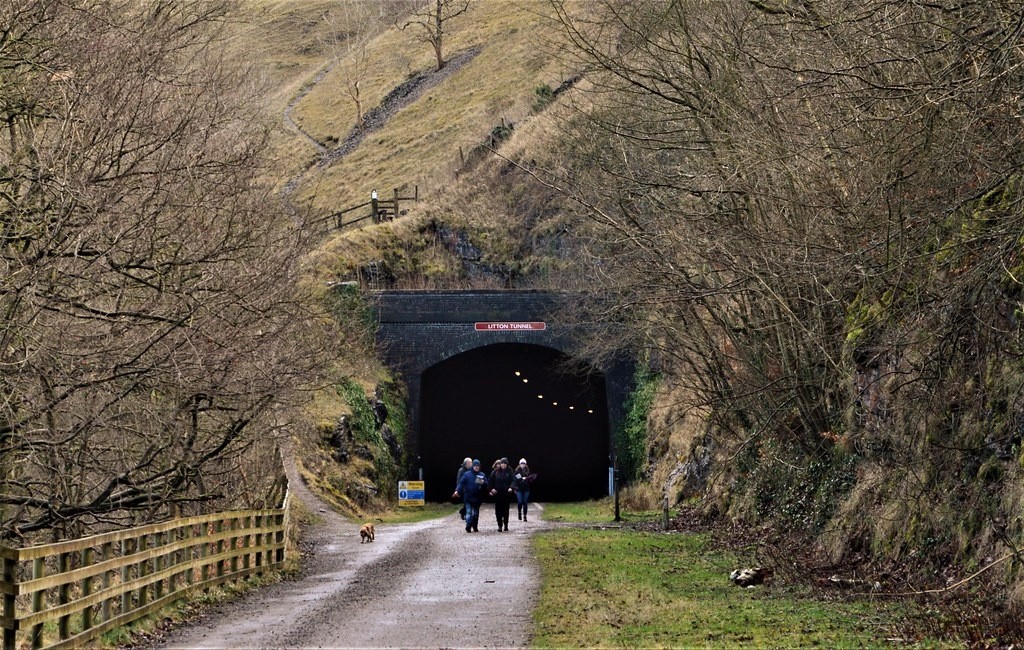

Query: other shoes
497;530;502;532
460;509;465;520
504;528;508;531
473;526;478;532
524;516;527;521
518;513;522;520
466;527;471;533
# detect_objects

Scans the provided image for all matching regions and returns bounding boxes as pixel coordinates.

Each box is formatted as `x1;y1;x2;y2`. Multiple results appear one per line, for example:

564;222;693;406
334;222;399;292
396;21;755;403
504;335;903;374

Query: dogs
360;523;376;543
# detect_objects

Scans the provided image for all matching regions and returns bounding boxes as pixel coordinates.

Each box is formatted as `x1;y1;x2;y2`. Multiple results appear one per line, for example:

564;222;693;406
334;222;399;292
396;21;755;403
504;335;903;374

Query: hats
519;458;527;464
472;459;480;467
500;458;508;464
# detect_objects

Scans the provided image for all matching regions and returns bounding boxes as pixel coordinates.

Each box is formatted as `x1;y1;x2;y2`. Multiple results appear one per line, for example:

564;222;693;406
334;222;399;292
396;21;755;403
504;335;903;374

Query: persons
452;458;489;533
514;458;534;522
487;457;515;532
456;457;473;520
